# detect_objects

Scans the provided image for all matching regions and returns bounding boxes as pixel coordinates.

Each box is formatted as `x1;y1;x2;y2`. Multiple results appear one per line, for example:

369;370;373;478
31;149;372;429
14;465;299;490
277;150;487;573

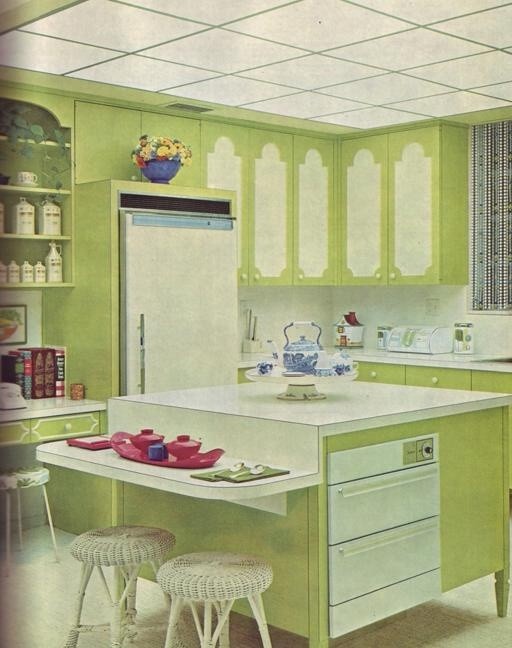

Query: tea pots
267;322;323;374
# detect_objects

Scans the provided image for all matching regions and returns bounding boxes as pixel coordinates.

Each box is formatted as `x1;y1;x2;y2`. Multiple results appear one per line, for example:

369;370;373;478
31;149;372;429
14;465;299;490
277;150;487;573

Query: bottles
0;242;63;283
454;323;473;354
377;326;392;350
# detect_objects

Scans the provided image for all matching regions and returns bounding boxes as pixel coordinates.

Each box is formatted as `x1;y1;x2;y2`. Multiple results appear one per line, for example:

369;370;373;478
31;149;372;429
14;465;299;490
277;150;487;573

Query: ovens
328;431;442;639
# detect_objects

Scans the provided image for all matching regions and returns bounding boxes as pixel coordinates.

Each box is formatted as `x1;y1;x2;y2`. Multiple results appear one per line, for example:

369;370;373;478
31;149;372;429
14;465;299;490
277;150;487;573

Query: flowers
131;135;193;168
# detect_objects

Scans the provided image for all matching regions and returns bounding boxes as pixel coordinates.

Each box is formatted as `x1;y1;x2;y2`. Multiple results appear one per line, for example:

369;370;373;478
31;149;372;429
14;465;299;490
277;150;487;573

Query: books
0;346;65;400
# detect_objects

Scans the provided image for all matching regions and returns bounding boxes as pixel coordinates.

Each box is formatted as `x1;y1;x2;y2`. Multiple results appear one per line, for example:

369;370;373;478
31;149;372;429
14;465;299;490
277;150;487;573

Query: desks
0;395;107;446
36;379;511;647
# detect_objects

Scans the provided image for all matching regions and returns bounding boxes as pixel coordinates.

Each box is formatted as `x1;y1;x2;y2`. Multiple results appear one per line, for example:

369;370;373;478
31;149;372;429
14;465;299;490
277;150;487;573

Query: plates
11;181;39;188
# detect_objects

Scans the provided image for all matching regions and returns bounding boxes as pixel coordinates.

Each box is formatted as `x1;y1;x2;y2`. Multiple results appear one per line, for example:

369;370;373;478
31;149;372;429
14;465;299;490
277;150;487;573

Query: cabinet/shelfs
354;361;405;385
471;371;512;396
294;133;338;287
74;99;201;187
0;85;76;288
338;119;469;286
201;120;294;286
406;365;471;392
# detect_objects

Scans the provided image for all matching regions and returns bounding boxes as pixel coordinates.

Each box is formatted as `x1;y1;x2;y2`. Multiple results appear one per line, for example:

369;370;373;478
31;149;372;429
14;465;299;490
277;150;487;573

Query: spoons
211;461;245;477
230;465;266;479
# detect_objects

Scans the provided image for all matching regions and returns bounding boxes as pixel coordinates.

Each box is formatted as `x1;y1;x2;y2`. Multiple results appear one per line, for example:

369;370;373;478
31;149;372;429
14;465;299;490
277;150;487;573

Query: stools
156;552;274;647
0;467;59;576
66;524;176;647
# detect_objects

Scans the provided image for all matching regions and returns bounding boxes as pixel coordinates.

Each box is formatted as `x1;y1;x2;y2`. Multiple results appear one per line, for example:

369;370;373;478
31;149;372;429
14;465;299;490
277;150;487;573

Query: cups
148;443;169;460
256;361;272;376
129;429;164;451
313;358;359;378
17;171;37;182
166;435;202;461
70;384;84;400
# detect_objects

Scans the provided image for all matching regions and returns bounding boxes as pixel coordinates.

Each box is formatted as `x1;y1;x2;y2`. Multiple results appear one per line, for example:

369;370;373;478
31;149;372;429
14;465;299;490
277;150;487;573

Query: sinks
468;357;512;363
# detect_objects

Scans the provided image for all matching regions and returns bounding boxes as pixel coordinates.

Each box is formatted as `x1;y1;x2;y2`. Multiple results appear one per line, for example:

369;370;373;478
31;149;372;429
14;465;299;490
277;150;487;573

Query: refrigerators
120;194;240;397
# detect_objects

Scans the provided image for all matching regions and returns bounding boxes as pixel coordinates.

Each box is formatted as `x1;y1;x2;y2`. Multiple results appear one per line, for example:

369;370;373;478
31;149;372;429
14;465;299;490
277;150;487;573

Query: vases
140;159;181;184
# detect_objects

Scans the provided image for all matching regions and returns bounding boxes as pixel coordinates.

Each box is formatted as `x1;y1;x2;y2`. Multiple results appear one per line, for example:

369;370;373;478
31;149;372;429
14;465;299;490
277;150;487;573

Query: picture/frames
0;305;27;345
